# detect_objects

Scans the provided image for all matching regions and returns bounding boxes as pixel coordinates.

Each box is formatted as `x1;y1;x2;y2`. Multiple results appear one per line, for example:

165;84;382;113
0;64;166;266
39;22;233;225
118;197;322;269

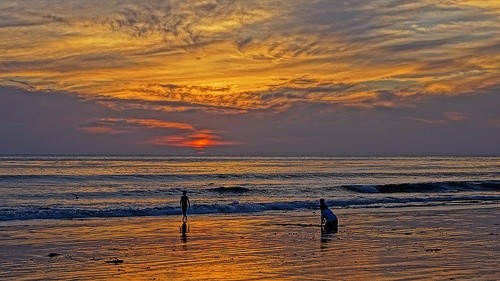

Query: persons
321;204;338;233
319;198;328;211
180;190;191;220
